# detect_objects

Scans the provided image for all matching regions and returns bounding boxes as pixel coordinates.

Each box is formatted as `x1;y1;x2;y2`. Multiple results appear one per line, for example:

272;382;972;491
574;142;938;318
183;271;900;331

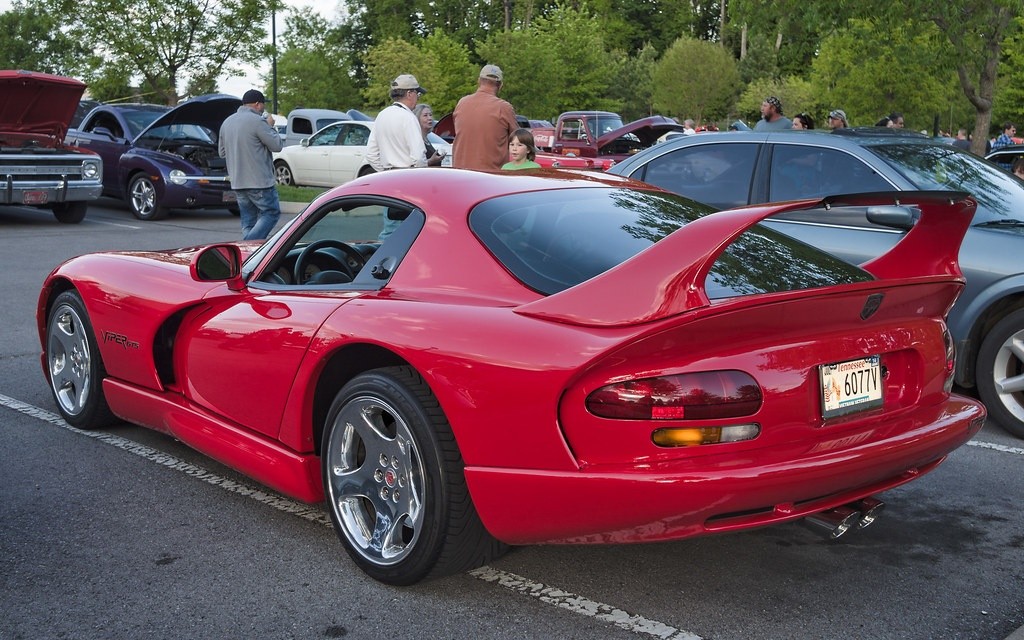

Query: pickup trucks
278;109;357;147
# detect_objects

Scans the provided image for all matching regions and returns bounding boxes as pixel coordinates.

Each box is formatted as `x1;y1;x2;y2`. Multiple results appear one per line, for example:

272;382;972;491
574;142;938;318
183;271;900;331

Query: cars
932;137;970;152
983;145;1024;172
605;129;1024;438
272;121;454;188
551;111;685;164
509;151;616;172
656;128;730;184
0;70;103;224
51;94;242;221
529;120;554;129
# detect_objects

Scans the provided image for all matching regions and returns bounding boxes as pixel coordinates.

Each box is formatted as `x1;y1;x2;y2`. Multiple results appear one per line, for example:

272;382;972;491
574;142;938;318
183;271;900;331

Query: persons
366;75;428;242
940;121;1024;180
753;97;791;130
218;90;283;241
790;113;816;130
827;109;848;129
500;129;542;170
452;65;522;169
412;103;447;167
875;112;906;128
664;117;696;135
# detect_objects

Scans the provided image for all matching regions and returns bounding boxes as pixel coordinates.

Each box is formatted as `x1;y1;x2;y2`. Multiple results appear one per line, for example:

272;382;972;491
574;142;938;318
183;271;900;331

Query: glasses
830;111;844;118
408;90;421;97
801;112;809;123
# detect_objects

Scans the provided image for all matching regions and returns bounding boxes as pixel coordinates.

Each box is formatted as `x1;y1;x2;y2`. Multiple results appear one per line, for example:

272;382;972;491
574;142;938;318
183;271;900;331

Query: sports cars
36;166;986;587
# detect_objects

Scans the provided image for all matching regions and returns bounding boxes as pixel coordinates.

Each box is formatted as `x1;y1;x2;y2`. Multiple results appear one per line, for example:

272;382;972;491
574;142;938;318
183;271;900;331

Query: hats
828;110;846;121
391;75;426;94
242;89;272;104
480;65;504;82
